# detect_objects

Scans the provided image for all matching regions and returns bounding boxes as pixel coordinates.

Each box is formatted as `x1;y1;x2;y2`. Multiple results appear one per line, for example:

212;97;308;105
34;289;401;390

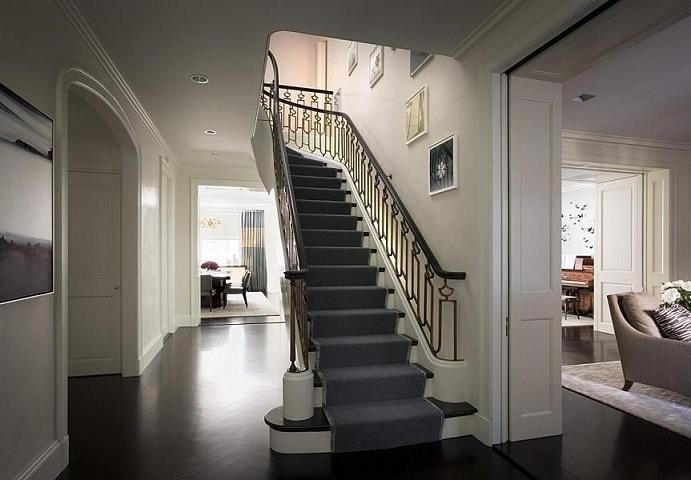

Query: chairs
201;270;251;311
607;290;691;395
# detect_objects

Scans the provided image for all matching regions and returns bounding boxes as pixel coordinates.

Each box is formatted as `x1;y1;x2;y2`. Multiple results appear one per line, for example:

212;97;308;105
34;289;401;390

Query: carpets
561;314;593;326
561;360;691;439
201;292;280;319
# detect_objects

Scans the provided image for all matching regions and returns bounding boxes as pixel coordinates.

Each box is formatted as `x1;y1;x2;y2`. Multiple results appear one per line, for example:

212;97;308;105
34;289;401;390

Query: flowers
201;261;218;271
660;280;691;307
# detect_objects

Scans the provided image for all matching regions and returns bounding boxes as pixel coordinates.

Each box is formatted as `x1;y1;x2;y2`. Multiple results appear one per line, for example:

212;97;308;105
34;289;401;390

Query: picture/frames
410;50;434;76
428;133;458;197
347;41;358;76
404;86;428;144
368;45;383;88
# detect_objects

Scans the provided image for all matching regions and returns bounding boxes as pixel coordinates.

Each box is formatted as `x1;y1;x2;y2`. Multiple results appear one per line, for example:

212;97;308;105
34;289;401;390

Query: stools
562;295;580;320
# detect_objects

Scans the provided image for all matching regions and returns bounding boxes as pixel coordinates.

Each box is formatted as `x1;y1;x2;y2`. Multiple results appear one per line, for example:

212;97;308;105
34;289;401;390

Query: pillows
649;302;691;342
621;291;662;338
